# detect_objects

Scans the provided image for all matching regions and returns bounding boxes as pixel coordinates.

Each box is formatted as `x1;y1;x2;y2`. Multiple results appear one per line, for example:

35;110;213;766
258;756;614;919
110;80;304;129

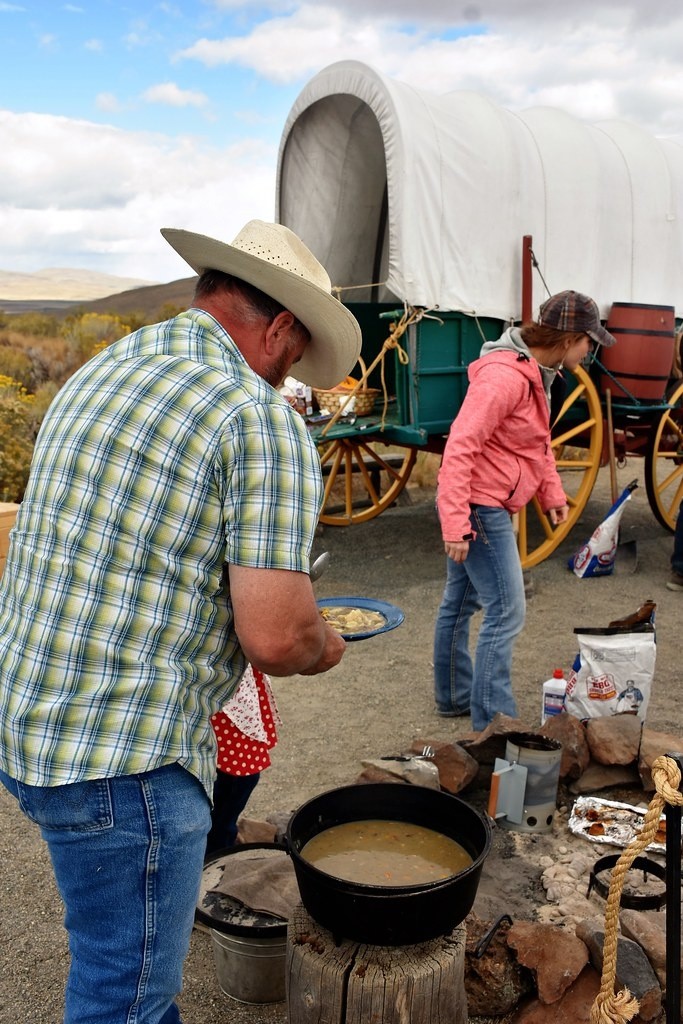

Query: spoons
308;550;330;584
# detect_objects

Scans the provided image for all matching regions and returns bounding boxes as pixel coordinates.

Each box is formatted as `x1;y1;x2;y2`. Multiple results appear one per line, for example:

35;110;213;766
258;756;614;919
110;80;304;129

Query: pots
275;778;497;947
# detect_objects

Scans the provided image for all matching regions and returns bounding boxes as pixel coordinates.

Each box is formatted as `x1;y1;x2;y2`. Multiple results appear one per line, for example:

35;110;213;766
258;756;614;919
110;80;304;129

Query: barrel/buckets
601;301;675;406
208;926;286;1004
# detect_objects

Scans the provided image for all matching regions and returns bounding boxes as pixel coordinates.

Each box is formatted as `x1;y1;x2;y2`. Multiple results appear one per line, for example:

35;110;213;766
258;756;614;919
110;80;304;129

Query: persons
209;666;283;867
0;219;362;1024
434;290;617;733
666;498;683;591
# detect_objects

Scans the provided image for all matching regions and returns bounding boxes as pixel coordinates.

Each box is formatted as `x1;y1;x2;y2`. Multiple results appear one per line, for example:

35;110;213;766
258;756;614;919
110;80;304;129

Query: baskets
313;355;381;416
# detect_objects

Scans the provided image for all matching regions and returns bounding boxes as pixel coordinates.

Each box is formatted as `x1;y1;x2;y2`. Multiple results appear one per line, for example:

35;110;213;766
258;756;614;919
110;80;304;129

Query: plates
315;597;406;641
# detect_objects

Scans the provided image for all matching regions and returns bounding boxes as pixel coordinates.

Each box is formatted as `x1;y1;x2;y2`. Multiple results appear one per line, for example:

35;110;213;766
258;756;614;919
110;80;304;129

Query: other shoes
666;572;682;592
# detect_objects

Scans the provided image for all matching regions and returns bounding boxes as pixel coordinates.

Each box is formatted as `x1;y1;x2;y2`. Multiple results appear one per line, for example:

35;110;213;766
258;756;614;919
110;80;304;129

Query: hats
538;290;616;347
161;219;362;390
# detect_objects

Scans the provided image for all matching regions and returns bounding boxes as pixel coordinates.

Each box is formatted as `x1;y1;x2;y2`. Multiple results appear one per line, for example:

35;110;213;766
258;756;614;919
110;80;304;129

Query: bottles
541;667;567;726
